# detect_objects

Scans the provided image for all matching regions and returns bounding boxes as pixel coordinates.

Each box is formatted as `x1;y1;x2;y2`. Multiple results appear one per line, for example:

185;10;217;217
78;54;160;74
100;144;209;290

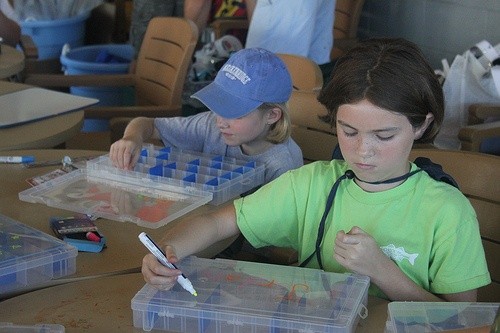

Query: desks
0;81;86;151
0;44;26;79
0;151;244;294
0;270;494;333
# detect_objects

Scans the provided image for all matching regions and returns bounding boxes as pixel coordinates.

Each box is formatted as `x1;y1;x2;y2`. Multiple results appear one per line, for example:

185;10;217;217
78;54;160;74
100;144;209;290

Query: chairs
456;101;500;151
211;0;366;63
410;148;500;303
26;17;200;150
244;53;342;264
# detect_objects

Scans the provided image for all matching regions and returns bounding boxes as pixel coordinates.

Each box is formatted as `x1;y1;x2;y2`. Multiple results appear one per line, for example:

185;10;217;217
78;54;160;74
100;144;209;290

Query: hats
190;46;292;120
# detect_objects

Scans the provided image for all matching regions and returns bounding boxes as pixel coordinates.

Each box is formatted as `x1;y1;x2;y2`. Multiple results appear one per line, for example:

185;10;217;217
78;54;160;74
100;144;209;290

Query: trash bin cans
60;43;138;132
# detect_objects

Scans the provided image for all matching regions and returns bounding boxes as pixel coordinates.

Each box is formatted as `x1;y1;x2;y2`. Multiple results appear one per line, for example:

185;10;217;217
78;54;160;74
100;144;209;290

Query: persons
108;47;304;261
131;0;214;49
141;38;491;303
245;0;335;69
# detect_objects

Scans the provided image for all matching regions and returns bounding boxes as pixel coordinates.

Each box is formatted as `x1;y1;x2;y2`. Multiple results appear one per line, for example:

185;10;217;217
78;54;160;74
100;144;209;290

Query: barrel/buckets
8;7;93;63
61;43;138;134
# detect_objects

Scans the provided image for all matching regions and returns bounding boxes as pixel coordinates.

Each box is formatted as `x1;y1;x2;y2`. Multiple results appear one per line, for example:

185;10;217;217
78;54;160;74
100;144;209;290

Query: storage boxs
131;255;372;333
17;142;266;230
0;214;78;297
384;301;500;333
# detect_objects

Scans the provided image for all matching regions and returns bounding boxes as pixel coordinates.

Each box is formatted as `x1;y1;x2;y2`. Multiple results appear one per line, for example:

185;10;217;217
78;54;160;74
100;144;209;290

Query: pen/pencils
0;156;35;163
138;231;198;297
